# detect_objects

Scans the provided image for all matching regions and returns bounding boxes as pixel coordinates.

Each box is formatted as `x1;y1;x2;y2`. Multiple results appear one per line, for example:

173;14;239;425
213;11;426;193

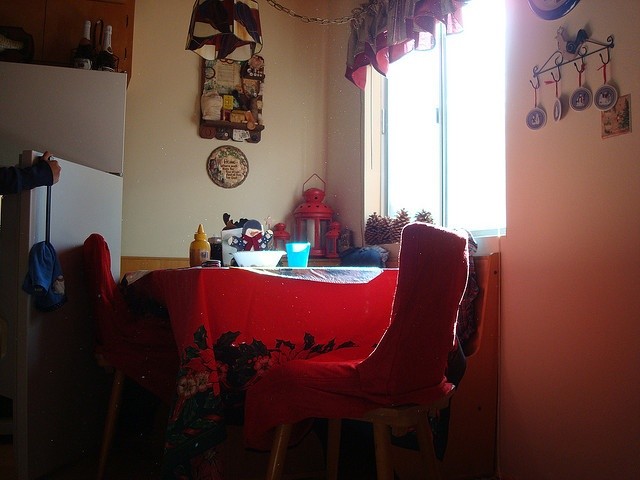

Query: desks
96;266;399;480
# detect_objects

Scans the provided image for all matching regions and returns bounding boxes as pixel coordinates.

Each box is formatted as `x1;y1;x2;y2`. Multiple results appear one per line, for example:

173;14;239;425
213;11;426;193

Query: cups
286;242;310;268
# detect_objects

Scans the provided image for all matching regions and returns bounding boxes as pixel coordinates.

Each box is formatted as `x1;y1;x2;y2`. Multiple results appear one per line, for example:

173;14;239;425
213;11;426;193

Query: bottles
98;24;116;72
189;223;211;267
77;19;94;71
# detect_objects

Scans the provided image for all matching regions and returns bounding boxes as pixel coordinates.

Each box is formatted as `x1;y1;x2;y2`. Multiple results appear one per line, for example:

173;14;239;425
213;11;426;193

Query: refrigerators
1;61;127;479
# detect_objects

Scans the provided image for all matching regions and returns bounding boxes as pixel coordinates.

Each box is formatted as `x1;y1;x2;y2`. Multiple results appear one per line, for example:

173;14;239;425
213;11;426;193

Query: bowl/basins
232;250;287;267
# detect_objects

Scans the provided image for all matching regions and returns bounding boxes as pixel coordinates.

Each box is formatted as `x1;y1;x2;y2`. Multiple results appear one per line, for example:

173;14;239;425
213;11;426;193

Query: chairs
248;223;470;480
